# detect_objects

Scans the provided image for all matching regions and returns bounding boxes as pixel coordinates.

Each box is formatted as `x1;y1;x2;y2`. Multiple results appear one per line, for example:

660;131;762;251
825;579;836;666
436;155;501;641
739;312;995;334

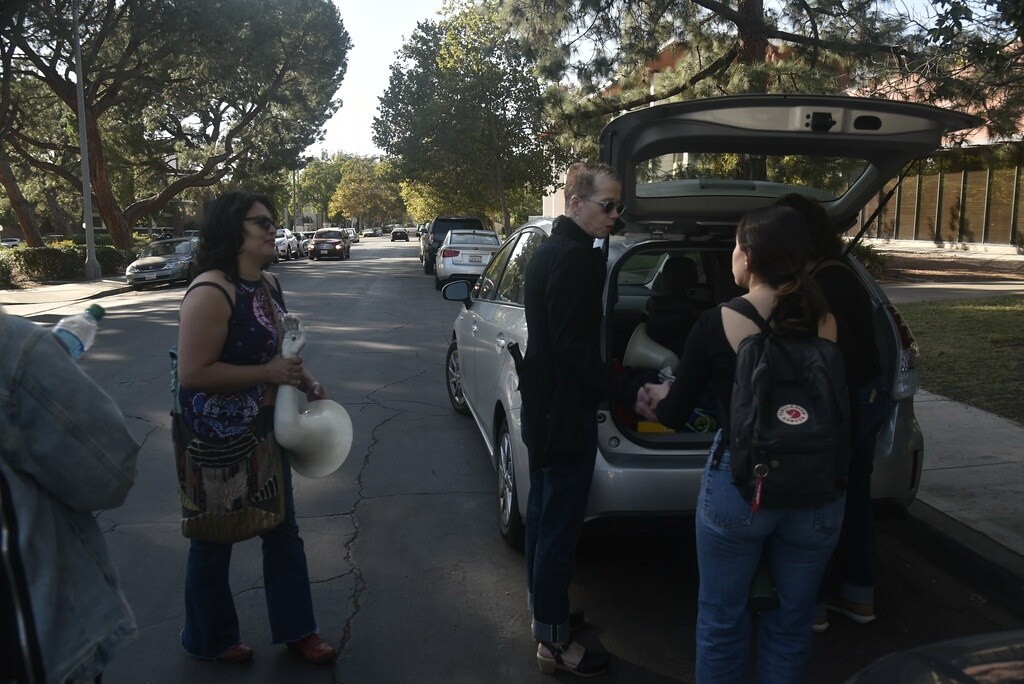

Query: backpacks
719;297;862;513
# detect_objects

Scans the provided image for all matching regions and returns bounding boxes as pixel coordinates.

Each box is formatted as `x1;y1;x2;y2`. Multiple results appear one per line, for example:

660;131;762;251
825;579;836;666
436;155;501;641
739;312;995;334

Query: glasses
578;197;626;216
246;214;276;230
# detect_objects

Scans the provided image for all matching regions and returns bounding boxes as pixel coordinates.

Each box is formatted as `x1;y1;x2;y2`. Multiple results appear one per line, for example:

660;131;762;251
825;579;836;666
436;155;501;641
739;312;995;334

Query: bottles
52;304;106;362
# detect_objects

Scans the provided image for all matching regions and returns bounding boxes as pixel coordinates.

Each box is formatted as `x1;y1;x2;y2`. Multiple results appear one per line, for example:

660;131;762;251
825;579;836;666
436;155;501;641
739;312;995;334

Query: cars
345;228;359;243
292;232;309;256
125;237;200;291
384;224;402;233
390;228;409;241
362;227;382;237
275;227;300;260
303;231;316;239
184;230;200;237
1;238;21;248
434;230;504;289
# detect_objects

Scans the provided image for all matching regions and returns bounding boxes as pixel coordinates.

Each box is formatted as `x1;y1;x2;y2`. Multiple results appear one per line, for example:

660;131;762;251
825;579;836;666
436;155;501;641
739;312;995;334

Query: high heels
568;610;585;632
536;636;611;676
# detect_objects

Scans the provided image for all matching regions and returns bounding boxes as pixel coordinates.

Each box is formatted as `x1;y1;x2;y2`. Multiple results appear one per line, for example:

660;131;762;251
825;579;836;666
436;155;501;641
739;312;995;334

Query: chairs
646;257;728;340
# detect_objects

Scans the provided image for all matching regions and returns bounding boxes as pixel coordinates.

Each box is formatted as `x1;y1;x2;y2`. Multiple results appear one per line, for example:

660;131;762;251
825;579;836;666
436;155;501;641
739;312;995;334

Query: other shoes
812;614;829;632
826;592;876;623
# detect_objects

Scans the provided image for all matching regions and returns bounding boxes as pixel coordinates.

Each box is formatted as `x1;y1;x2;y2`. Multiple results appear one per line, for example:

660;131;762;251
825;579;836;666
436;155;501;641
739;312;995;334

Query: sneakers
218;642;254;660
285;633;335;661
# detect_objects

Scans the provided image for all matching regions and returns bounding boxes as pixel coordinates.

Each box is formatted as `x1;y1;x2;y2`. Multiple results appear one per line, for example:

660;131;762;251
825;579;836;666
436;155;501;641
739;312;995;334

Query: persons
0;304;141;684
645;192;880;684
169;191;336;665
518;163;658;679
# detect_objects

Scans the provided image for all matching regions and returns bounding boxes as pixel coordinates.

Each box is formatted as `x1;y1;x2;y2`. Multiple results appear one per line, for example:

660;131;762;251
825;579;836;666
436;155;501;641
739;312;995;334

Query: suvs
443;95;987;556
417;219;486;273
308;227;351;259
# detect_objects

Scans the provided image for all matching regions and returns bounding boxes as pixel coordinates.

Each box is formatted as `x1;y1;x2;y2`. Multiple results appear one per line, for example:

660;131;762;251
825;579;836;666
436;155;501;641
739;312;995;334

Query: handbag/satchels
170;404;285;544
